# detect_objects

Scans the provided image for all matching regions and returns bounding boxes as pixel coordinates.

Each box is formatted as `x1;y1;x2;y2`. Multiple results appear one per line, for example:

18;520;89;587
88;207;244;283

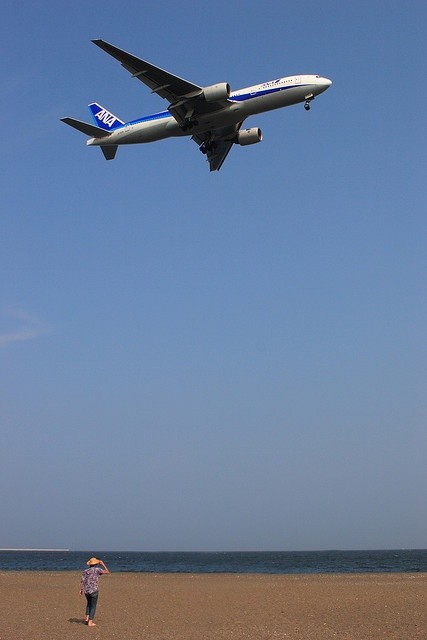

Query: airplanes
58;37;332;173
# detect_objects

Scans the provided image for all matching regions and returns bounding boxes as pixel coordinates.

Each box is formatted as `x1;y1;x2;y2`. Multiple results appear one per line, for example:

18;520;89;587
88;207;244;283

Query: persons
79;558;109;627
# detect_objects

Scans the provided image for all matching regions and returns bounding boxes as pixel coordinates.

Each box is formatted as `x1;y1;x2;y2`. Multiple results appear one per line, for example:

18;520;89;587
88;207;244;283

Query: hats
87;558;100;565
